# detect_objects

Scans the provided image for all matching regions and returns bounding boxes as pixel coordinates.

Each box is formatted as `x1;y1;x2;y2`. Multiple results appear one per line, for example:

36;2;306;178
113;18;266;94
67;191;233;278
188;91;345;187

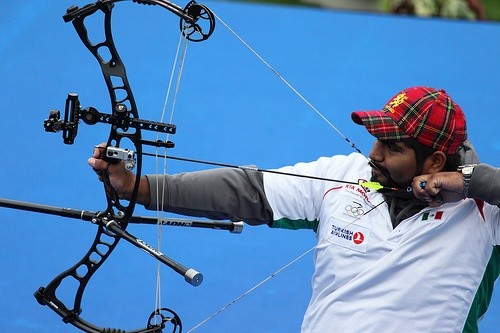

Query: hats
351;86;468;156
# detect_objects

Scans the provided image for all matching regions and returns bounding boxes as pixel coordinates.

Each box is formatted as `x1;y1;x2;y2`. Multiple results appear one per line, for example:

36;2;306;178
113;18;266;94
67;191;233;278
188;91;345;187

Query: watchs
460;164;474;200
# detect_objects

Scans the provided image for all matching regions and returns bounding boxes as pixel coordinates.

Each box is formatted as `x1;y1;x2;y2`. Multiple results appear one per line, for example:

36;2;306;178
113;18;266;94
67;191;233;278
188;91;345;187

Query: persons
89;86;500;332
